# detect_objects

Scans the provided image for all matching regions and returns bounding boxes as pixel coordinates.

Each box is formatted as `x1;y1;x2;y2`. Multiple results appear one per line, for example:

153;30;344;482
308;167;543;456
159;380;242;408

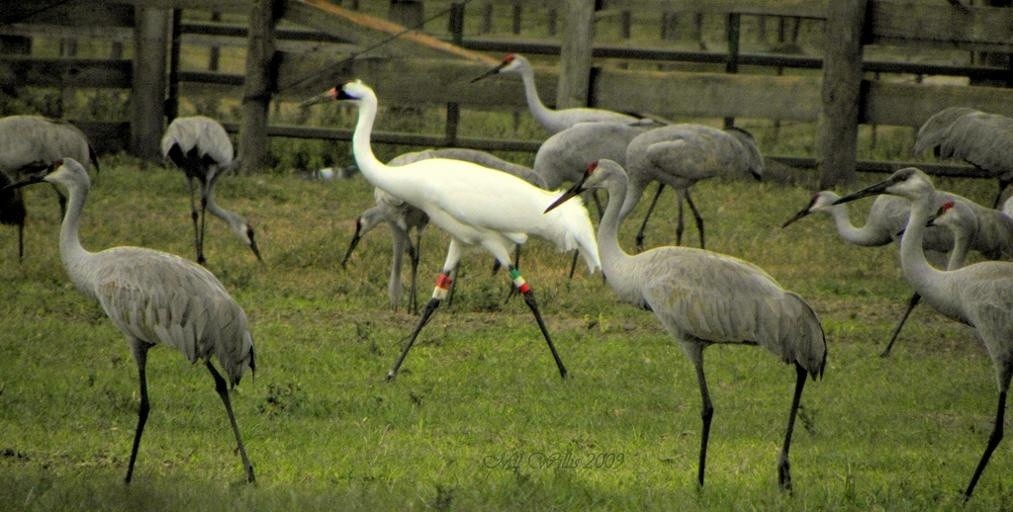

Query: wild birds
159;113;264;267
781;107;1013;507
340;48;768;318
296;79;603;384
0;113;100;266
542;159;827;501
0;157;260;490
